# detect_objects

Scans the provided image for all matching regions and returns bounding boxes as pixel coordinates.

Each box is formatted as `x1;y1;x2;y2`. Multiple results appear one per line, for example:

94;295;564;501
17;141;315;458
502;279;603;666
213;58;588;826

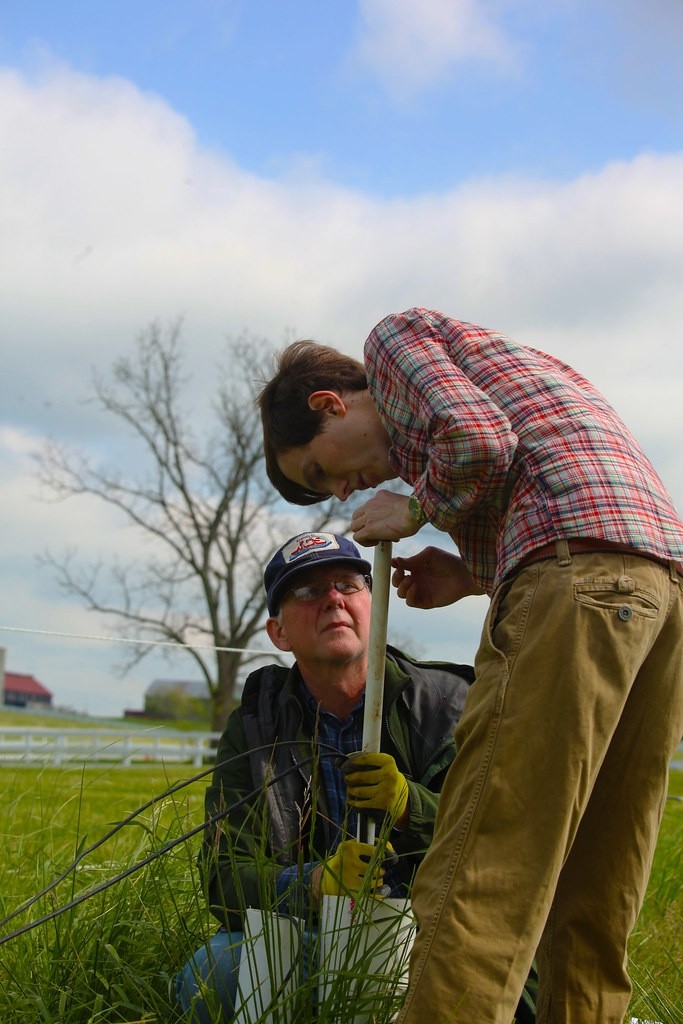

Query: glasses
282;571;371;601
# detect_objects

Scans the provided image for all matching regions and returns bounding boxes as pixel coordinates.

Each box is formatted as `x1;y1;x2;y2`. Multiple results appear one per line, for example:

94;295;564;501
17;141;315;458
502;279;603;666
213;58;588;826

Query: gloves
335;751;411;830
321;838;398;898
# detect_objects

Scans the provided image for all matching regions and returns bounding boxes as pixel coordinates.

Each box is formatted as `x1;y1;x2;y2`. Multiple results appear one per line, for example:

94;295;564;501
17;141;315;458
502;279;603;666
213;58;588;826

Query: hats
263;531;372;618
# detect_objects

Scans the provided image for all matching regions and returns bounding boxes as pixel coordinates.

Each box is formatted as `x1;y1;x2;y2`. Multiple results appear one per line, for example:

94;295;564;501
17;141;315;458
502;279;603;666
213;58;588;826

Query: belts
511;537;683;579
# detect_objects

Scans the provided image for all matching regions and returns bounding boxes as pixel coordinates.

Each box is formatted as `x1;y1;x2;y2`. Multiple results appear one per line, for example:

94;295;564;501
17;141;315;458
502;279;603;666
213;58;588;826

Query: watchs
408;494;427;526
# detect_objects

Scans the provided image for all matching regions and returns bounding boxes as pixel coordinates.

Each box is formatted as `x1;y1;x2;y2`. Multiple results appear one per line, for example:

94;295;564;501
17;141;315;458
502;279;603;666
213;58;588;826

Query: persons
252;309;683;1024
173;533;538;1024
